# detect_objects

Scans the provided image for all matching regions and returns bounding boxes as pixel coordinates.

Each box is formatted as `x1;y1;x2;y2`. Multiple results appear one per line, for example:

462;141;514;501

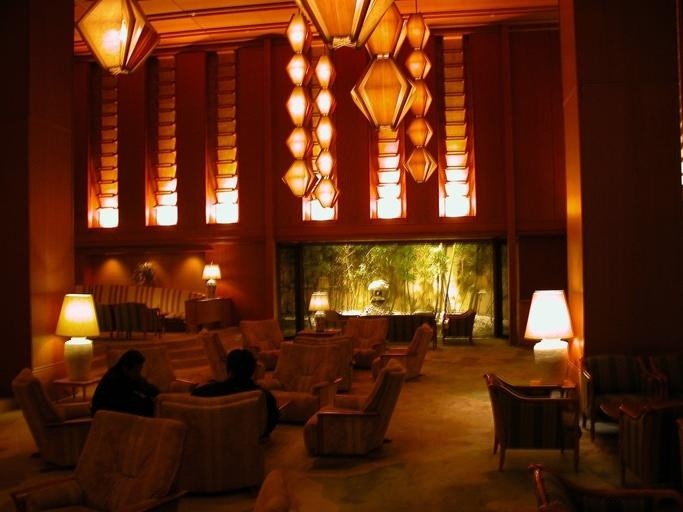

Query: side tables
297;326;342;338
53;377;101;400
530;377;576;399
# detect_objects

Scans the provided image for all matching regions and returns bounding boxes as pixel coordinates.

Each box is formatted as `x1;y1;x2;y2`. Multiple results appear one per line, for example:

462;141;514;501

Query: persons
90;349;159;419
193;350;281;444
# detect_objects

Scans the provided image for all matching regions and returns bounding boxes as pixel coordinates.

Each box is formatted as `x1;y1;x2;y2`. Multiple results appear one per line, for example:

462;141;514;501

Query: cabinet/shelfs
195;297;233;329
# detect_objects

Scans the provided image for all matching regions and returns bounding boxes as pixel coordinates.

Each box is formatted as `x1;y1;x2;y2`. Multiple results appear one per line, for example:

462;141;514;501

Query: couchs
75;283;206;332
12;410;189;511
442;308;477;341
265;341;341;413
303;358;408;458
153;390;266;499
527;463;683;512
94;302;168;341
200;330;265;381
12;368;93;473
294;335;354;391
239;320;294;369
616;399;683;489
372;323;434;381
254;469;291;512
484;371;581;474
107;345;198;396
650;351;683;401
336;311;438;350
578;353;663;442
342;317;389;369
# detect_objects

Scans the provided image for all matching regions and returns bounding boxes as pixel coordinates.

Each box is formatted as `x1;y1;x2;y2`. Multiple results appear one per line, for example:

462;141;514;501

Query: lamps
308;291;331;332
404;11;440;182
76;0;160;76
295;0;395;49
55;294;101;381
201;260;222;298
283;7;318;197
523;290;574;385
313;39;341;208
350;0;417;130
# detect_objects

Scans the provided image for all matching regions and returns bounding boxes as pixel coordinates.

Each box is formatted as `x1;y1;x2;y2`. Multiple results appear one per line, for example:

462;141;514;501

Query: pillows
27;479;82;510
257;341;268;351
274;369;301;391
352;351;372;371
279;406;309;424
149;354;175;392
263;356;276;371
